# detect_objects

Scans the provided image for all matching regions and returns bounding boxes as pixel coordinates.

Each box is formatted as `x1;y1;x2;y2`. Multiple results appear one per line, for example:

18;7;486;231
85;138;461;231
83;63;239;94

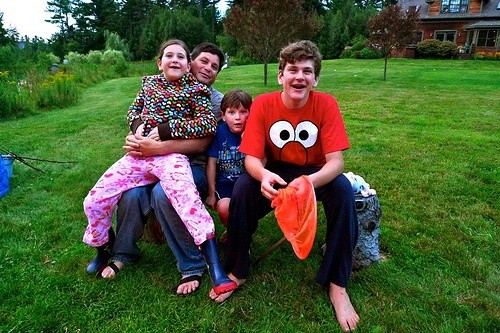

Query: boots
200;235;237;293
85;226;114;275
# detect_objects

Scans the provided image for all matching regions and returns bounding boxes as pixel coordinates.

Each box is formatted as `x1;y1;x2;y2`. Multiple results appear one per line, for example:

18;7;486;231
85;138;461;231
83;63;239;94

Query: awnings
466;21;500;27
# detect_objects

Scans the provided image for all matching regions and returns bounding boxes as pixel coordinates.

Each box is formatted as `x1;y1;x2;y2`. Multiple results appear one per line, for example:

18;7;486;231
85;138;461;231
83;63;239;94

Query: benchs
142;172;383;271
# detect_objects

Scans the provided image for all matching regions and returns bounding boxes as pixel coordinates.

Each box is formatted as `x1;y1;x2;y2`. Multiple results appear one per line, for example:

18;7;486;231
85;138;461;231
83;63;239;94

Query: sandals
98;263;119;279
175;275;202;296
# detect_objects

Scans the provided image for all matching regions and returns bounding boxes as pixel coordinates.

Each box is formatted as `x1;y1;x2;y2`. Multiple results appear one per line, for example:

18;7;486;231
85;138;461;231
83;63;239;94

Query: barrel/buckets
2;155;15;178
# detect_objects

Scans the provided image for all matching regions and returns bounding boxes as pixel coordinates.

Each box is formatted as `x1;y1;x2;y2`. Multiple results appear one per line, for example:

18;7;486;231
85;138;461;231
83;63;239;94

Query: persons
97;41;225;295
198;88;252;244
457;41;472;54
83;40;238;295
209;40;360;332
